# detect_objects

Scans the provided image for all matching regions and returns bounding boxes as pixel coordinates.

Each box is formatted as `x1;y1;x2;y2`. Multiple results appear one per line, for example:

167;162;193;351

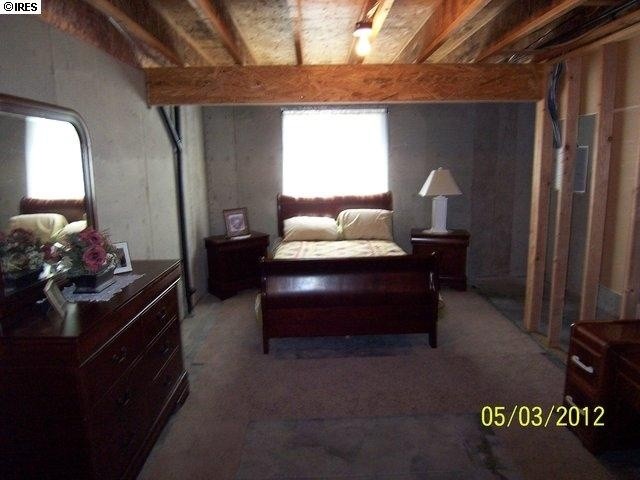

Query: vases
67;262;117;294
1;264;45;288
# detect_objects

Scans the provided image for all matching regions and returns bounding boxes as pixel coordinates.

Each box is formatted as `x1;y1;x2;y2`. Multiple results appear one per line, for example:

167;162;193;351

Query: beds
257;189;440;356
17;194;88;223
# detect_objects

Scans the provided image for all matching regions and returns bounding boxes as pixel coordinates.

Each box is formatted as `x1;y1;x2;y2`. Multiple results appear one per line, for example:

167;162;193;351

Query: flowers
0;227;62;279
57;223;119;277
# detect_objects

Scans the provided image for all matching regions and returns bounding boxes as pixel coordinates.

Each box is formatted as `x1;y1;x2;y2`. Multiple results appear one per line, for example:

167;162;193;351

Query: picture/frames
42;277;69;319
223;207;251;240
111;241;134;276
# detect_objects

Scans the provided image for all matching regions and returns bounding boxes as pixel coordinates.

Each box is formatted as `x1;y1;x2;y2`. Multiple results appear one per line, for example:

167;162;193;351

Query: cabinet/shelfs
0;261;191;480
562;315;640;457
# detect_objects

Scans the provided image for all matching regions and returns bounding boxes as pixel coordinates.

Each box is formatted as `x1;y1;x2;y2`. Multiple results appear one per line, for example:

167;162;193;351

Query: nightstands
409;226;471;292
204;230;270;301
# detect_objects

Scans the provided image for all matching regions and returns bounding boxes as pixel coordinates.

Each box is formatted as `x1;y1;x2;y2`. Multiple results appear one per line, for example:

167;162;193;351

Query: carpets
141;288;615;480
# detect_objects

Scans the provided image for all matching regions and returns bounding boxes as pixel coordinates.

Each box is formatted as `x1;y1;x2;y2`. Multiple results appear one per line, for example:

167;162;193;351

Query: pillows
8;213;68;246
336;208;394;242
282;215;343;243
48;219;88;247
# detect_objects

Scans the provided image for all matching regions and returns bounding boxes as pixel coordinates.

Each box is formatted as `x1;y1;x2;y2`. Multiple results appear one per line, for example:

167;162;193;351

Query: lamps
416;166;464;235
351;22;373;58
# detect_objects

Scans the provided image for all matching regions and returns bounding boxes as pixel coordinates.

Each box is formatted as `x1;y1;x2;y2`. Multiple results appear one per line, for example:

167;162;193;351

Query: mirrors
0;91;99;319
